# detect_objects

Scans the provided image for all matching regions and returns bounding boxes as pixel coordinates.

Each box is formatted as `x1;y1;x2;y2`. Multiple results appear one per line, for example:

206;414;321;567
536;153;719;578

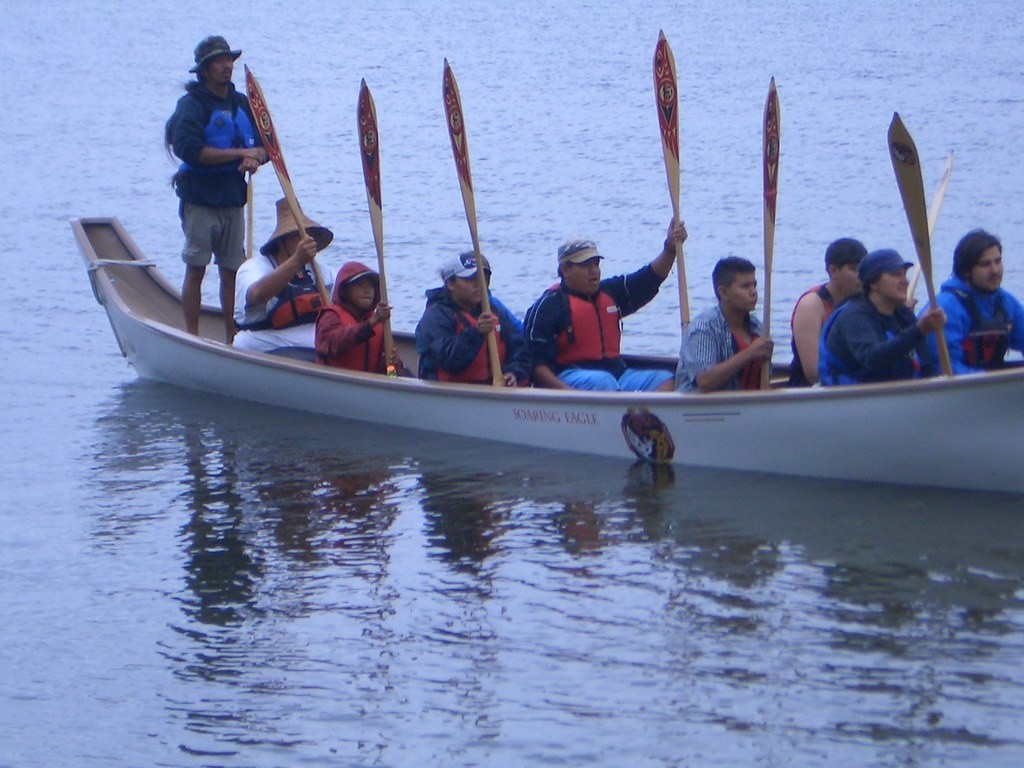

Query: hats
260;196;334;255
188;36;242;73
443;251;477;282
858;248;913;281
557;239;605;265
480;254;492;276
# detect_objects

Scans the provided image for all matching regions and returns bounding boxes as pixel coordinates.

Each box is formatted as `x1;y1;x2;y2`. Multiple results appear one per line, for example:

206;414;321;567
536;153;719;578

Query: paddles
247;168;253;260
440;56;506;386
653;28;694;330
887;110;956;373
356;77;394;369
243;62;330;307
756;77;783;390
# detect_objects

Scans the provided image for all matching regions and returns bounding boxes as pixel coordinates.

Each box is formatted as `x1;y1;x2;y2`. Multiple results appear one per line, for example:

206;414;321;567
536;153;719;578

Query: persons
788;238;918;386
232;196;333;365
172;36;271;343
818;249;948;386
914;231;1024;380
315;262;412;377
674;256;774;392
415;251;534;387
523;217;688;392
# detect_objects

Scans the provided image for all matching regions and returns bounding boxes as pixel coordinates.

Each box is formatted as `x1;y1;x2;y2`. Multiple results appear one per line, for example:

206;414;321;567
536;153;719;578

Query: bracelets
664;239;675;253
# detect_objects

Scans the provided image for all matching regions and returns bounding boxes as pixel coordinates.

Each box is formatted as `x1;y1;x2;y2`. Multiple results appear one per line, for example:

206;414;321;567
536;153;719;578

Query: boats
71;216;1024;497
